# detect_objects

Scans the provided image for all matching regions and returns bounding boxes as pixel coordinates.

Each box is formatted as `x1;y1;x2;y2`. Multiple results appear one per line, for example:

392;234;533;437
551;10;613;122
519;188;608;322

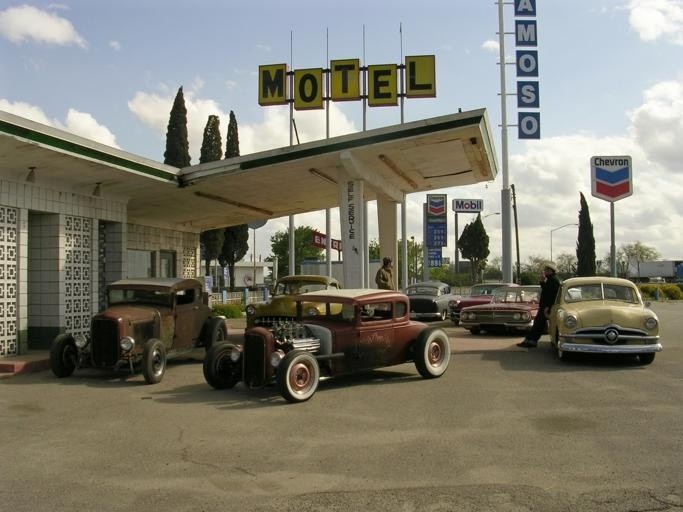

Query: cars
52;277;230;383
548;276;663;366
204;286;452;404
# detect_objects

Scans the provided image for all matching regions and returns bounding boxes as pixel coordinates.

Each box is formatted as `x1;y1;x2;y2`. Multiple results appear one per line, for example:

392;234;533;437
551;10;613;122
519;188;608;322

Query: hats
382;256;392;264
544;261;560;274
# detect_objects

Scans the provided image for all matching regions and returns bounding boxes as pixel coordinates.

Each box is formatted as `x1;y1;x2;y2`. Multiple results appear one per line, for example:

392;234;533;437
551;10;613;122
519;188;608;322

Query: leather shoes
516;341;528;347
523;342;537;347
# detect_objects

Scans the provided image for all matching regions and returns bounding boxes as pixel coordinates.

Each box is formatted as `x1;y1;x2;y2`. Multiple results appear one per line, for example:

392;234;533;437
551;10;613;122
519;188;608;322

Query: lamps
25;167;37;183
92;182;103;196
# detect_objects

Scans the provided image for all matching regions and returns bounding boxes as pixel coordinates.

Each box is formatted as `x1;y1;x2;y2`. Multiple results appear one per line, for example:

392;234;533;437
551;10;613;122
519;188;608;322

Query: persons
515;265;560;349
374;256;395;311
